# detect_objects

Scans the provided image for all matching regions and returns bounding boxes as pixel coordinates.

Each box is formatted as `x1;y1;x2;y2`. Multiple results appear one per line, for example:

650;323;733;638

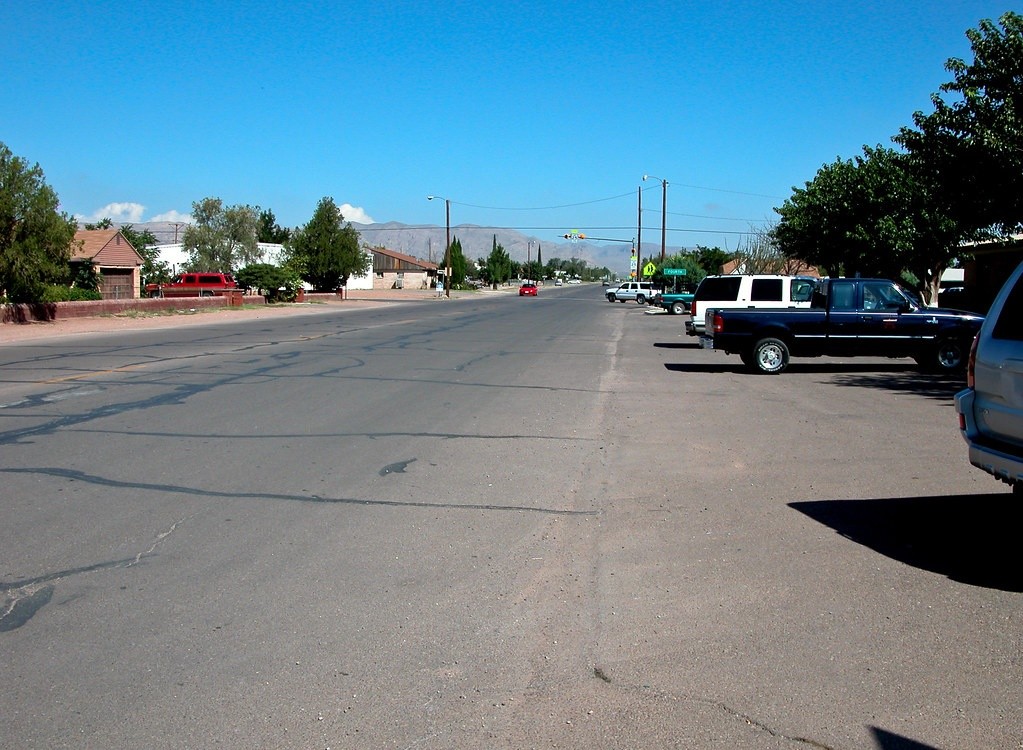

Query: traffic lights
564;234;584;239
632;248;635;253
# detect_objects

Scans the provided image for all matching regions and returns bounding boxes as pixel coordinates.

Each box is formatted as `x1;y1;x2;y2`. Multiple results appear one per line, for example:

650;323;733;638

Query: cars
519;284;538;297
602;280;610;285
555;280;582;286
466;278;485;289
616;278;624;282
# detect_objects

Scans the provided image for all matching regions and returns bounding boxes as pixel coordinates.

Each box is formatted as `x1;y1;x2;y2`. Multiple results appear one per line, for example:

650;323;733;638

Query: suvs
605;282;660;305
953;256;1023;494
145;273;235;298
685;274;820;341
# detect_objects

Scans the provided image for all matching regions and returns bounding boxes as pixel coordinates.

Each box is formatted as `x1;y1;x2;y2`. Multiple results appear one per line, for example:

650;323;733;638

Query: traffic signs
663;269;687;276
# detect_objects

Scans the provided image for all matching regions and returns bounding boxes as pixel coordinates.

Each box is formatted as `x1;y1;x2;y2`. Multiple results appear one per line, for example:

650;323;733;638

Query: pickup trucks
699;279;986;375
655;283;699;315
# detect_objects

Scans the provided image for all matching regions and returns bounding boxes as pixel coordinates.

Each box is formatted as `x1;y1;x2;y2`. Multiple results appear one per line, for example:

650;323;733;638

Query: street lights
428;196;450;297
643;175;666;262
527;239;536;285
